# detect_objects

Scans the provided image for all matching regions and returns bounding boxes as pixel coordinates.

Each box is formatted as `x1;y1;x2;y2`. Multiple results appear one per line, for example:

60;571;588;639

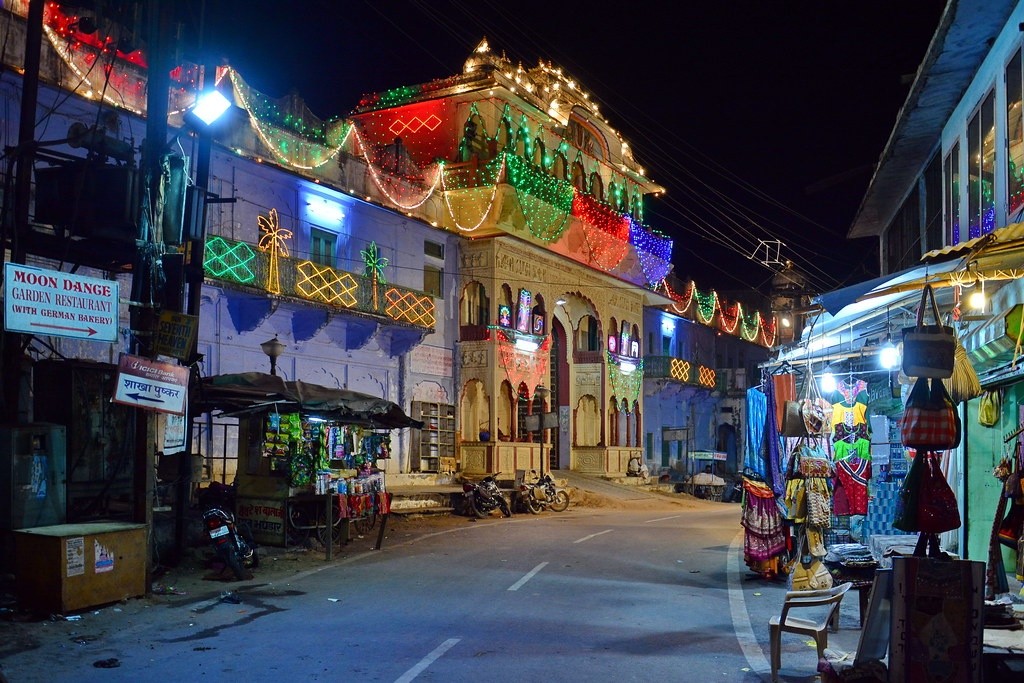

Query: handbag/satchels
902;285;956;377
888;533;986;681
901;377;962;451
893;450;961;531
777;373;835;591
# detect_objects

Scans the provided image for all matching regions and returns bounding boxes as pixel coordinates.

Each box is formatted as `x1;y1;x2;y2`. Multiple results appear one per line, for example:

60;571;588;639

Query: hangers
837;370;863;463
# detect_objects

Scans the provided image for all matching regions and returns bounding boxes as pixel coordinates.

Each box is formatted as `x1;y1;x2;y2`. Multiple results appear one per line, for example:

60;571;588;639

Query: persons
688;484;724;499
701;464;712;474
626;451;649;479
335;444;354;469
660;474;674;483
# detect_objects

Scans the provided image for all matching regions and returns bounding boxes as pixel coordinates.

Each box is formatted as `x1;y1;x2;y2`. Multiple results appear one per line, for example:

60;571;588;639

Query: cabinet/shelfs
15;523;148;612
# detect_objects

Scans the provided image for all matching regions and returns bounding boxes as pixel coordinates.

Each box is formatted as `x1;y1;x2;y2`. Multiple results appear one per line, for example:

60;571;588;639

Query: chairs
768;581;853;683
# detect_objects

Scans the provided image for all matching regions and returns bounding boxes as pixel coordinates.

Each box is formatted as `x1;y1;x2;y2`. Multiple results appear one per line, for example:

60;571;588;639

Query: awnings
201;370;425;432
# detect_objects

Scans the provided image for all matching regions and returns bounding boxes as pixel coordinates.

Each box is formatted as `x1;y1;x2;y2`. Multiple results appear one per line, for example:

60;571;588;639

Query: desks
869;534;960;569
832;579;872;631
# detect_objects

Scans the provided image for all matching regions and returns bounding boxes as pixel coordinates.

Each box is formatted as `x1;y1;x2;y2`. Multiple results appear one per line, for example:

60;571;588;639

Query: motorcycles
185;474;259;580
519;469;570;516
465;471;513;518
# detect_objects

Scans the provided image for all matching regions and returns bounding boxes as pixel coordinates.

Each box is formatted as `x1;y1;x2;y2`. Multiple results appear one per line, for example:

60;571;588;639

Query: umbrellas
687;472;726;490
